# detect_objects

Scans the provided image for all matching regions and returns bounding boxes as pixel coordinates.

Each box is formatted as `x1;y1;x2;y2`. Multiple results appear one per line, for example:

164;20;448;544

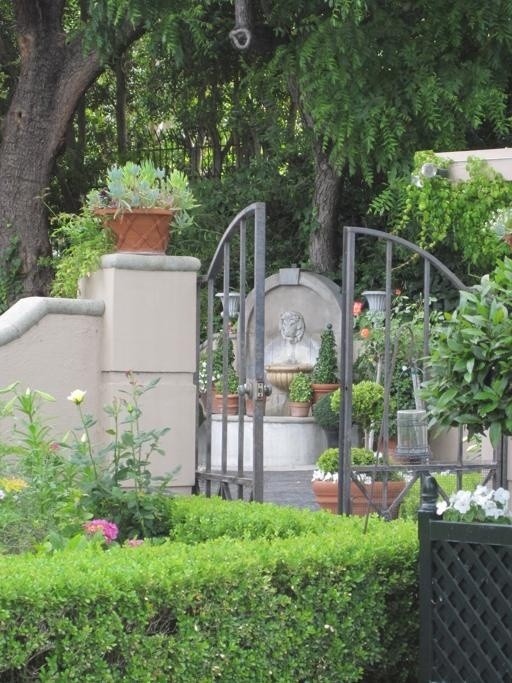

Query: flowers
81;160;198;229
431;480;510;526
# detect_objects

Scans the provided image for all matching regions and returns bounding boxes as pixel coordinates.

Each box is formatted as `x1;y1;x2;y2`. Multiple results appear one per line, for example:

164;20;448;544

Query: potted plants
357;259;392;333
202;331;241;415
287;322;341;417
312;443;407;520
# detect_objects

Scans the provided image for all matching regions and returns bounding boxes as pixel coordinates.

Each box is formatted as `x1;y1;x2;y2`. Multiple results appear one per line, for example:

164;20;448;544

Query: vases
94;206;175;254
